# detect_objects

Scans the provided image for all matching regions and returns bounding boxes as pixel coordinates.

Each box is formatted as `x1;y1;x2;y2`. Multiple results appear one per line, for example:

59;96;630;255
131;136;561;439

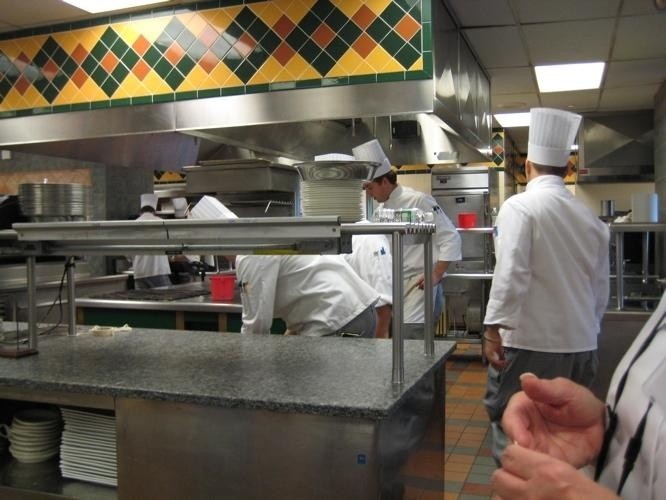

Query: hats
525;106;583;168
186;194;238;220
139;192;159;211
352;139;393;182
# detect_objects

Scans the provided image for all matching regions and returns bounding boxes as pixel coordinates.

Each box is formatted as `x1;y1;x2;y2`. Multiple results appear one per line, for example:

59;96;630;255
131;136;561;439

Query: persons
482;107;609;470
170;197;214;267
125;194;173;290
352;138;463;338
488;264;665;499
187;194;379;338
341;219;393;338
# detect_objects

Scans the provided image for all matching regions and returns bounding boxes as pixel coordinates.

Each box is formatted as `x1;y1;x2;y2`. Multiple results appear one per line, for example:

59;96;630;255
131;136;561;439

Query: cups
458;212;476;229
601;200;615;217
208;274;237;300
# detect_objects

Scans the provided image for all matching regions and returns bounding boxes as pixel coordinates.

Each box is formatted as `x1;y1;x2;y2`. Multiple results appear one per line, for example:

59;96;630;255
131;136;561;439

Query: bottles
378;207;433;225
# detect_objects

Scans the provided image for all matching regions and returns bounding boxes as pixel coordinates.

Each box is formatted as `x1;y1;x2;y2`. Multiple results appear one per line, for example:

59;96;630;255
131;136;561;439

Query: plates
630;193;659;224
7;409;118;489
296;180;366;222
17;180;84;221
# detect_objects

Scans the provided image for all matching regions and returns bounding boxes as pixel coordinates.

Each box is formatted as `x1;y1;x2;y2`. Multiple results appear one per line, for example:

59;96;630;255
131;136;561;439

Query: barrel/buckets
208;273;238;302
458;213;477;228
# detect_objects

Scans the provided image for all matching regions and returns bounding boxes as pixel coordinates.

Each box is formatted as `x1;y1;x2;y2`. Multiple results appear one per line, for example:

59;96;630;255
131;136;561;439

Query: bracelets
482;334;501;343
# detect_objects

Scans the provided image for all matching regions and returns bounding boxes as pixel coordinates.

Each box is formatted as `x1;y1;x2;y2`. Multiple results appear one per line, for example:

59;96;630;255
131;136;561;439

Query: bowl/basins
290;161;382;181
